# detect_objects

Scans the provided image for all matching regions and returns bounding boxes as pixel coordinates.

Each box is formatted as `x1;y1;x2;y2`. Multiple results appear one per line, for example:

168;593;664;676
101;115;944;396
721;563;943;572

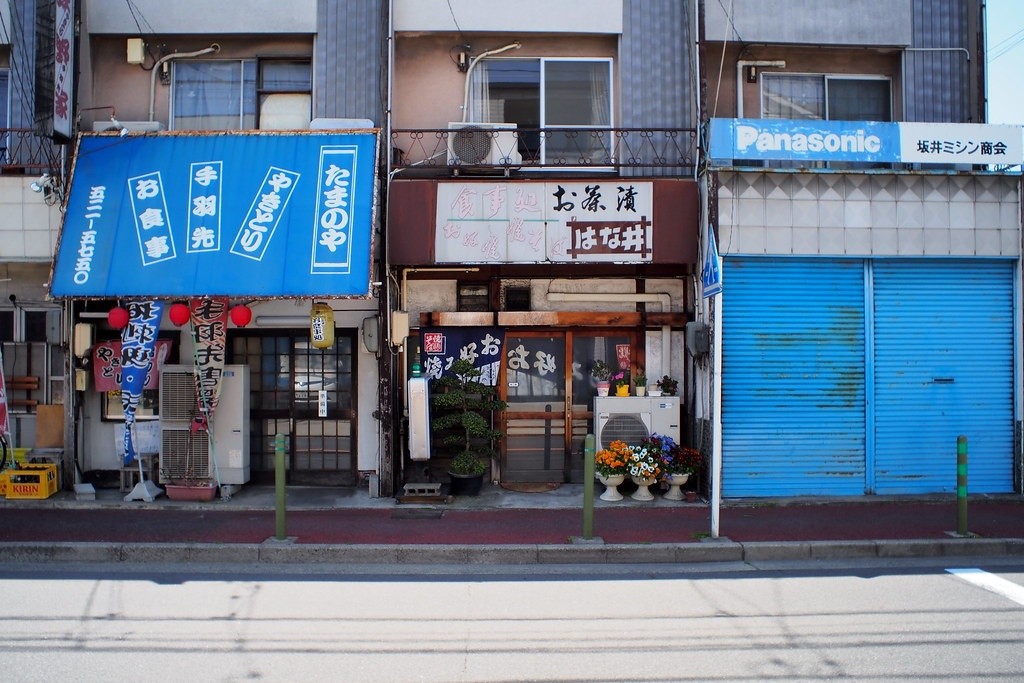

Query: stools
120;452;159;493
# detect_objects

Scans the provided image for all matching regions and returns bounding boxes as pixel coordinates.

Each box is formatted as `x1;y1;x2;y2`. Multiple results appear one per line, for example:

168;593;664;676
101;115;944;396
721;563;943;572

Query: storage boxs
0;447;64;500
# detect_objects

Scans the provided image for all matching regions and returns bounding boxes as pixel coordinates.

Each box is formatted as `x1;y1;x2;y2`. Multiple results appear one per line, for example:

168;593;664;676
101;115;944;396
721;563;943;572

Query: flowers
595;434;701;491
611;371;625;387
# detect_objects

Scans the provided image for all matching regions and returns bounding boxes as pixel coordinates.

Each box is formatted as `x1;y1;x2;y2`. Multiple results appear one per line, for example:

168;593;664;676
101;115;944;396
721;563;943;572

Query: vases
615;385;630;396
599;473;624;501
631;476;655;500
664;473;689;500
647;391;664;397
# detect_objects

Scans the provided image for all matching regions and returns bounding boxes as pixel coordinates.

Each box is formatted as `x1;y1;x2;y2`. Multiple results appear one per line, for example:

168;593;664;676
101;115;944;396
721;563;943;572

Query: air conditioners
447;122;518;167
93;120;167;131
158;364;250;485
712;158;770;168
913;163;972;171
593;396;681;456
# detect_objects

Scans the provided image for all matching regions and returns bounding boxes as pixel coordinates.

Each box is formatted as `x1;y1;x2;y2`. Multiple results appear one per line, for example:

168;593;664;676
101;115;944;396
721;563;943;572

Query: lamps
80;312;109;318
30;173;50;192
256;315;312;328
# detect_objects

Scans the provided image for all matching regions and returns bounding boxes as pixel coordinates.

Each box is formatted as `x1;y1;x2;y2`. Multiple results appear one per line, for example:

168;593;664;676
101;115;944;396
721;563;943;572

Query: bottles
9;465;53;483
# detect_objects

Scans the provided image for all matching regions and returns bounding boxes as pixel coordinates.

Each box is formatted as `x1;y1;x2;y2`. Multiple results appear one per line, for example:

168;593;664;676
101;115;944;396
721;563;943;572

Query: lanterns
310;302;334;350
169;303;191;328
230;304;252;327
107;307;128;331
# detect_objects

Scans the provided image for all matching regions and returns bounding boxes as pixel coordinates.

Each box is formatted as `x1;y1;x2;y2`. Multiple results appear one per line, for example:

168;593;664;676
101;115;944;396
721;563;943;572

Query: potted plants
633;376;648;397
656;375;678;396
158;461;218;501
430;359;510;497
589;359;615;396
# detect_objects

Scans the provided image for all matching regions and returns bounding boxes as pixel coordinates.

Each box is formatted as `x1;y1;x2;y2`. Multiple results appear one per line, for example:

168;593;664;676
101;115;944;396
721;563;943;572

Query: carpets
500;482;559;493
